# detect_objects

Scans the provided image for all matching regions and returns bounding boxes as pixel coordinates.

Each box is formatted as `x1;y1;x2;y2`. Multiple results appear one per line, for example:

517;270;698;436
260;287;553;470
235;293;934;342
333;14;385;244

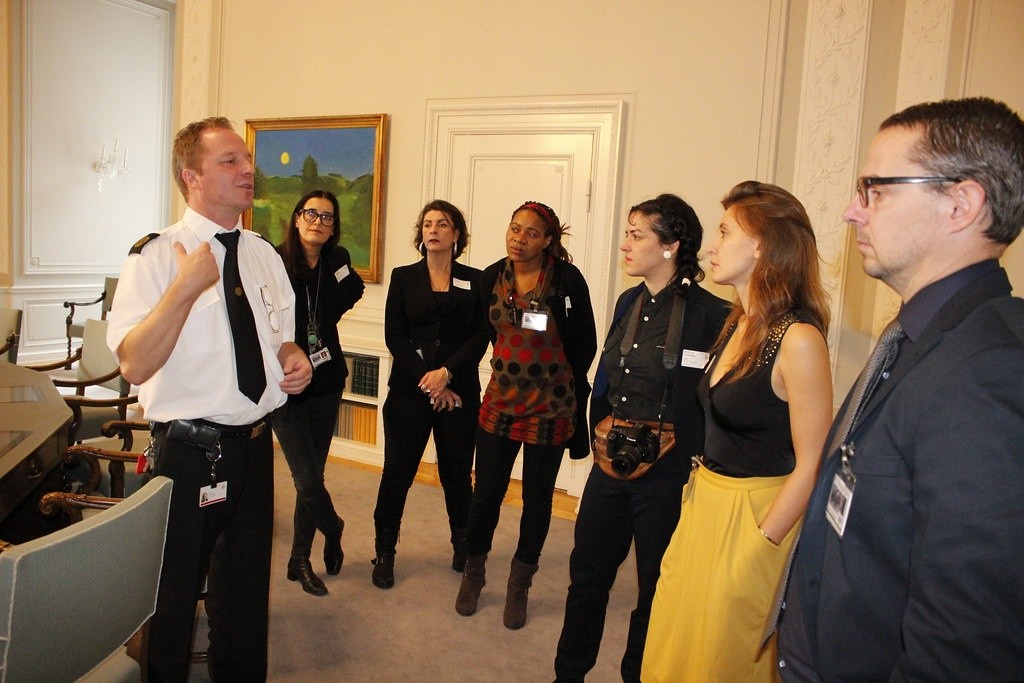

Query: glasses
298;209;335;227
857;176;963;208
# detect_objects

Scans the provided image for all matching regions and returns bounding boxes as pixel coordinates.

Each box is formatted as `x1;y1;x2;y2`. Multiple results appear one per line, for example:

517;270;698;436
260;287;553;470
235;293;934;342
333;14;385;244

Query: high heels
324;517;344;576
287;558;327;596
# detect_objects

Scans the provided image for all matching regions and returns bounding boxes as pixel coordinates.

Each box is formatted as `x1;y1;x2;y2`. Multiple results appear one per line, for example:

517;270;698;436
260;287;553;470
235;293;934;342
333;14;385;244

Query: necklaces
431;279;449;291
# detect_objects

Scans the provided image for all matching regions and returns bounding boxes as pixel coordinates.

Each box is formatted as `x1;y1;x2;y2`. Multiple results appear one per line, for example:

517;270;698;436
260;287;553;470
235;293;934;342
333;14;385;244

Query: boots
503;557;539;630
455;553;486;616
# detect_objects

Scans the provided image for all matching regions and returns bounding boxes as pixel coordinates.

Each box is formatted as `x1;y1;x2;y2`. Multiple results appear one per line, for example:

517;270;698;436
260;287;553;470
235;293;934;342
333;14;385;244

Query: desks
0;356;75;531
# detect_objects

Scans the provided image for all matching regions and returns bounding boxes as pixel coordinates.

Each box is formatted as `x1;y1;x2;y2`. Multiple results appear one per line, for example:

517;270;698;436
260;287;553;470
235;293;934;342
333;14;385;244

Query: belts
200;418;268;440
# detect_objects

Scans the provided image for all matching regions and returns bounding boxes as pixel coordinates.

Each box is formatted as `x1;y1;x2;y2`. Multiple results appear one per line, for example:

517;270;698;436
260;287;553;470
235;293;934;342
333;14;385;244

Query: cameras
606;423;660;477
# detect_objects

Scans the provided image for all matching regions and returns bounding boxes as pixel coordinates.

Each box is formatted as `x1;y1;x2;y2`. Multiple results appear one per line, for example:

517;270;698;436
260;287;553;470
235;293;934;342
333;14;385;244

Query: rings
421;384;427;391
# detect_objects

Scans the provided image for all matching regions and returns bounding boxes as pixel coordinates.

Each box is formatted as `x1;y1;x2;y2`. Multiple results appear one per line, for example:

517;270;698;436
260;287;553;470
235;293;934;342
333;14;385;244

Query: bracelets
758;526;779;546
445;368;451;383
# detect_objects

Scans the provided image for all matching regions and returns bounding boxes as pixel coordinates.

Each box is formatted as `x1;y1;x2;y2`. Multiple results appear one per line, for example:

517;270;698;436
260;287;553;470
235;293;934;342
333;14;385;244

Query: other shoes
371;551;394;588
452;553;465;572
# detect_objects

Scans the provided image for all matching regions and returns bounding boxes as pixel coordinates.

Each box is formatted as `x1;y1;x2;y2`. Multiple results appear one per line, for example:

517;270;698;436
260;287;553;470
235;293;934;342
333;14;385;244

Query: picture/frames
241;114;387;286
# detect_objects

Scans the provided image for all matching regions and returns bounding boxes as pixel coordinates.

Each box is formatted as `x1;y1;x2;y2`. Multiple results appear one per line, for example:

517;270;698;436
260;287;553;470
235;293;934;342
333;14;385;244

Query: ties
214;229;267;405
754;317;908;664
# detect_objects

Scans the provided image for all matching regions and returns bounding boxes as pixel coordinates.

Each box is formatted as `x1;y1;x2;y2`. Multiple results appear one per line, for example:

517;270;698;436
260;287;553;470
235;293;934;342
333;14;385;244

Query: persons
755;96;1024;683
370;200;492;589
638;180;833;683
322;353;327;358
456;201;597;628
552;193;733;683
202;493;208;502
274;190;365;595
105;116;312;683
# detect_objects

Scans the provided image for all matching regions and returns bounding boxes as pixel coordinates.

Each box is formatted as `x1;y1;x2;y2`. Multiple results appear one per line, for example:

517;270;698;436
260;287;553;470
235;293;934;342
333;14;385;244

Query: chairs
0;279;212;683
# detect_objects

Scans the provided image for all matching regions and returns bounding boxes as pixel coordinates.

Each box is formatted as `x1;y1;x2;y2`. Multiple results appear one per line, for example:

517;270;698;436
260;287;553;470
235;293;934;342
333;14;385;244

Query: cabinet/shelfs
328;345;393;470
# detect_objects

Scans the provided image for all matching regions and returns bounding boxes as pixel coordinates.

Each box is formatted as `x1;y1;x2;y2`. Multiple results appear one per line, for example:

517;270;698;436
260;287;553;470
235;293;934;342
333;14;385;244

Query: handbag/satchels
592;415;676;480
167;418;221;451
